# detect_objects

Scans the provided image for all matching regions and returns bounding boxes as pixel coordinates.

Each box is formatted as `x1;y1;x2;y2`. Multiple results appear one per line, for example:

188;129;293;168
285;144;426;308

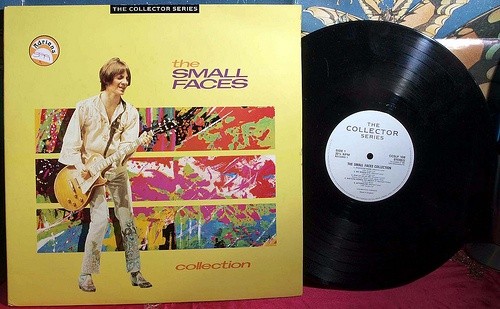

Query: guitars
54;117;176;211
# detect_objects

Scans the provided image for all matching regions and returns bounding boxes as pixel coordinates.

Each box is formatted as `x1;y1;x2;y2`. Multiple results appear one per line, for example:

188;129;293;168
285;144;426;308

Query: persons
58;57;153;293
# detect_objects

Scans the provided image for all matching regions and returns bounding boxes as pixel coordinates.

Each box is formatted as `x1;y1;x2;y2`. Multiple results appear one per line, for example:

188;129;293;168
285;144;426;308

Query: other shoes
79;274;96;292
131;271;152;288
